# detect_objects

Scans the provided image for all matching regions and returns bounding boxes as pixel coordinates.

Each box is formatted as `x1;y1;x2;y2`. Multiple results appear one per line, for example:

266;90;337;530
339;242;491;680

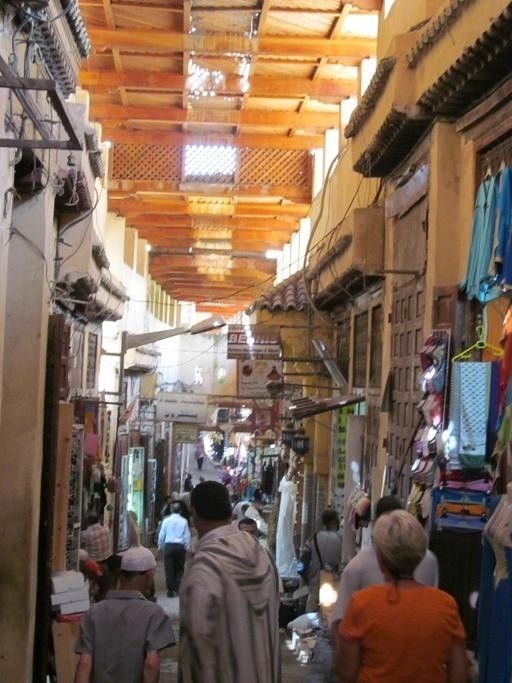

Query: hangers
483;144;506;181
451;325;505;362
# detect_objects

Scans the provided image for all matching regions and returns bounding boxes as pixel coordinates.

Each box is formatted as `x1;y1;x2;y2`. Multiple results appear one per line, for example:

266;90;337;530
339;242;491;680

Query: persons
80;442;258;602
328;497;440;649
177;481;282;683
74;546;176;683
333;509;470;683
305;507;343;613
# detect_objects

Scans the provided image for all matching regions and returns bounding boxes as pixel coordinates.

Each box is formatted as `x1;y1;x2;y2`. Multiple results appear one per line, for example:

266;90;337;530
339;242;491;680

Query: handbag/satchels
320;562;340;582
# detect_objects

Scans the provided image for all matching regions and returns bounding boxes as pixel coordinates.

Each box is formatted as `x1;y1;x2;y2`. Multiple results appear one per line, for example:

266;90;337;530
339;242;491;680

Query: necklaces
386;577;415;583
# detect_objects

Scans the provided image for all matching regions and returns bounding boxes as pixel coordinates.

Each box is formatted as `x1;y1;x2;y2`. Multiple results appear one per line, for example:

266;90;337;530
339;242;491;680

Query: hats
120;546;156;572
